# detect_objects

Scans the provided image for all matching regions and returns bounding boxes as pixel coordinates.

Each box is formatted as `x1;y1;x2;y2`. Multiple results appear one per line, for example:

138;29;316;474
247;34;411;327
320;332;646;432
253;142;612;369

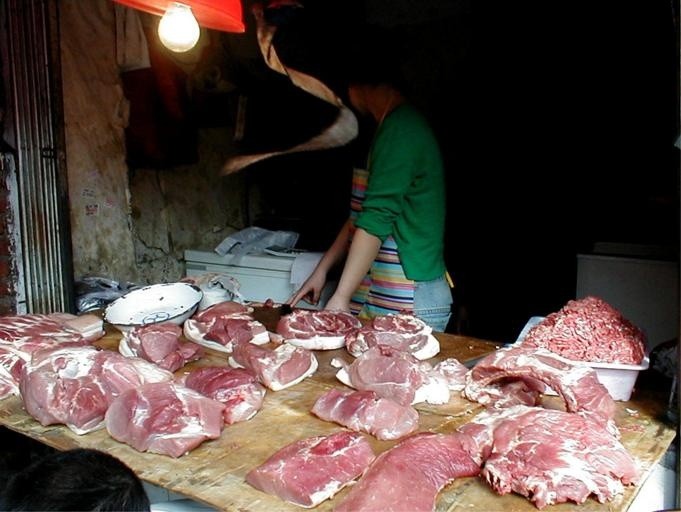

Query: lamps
115;0;247;52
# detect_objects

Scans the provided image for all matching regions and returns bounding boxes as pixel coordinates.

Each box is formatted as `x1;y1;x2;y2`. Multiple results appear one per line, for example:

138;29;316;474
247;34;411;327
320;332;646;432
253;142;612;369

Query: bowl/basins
101;282;205;334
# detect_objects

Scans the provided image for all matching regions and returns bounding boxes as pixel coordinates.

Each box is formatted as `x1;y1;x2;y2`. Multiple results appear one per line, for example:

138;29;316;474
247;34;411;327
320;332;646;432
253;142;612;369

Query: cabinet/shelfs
575;255;681;355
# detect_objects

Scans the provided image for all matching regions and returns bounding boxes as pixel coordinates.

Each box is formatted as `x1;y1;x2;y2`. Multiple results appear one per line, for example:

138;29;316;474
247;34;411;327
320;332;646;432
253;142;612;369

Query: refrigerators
573;240;679;368
179;241;345;312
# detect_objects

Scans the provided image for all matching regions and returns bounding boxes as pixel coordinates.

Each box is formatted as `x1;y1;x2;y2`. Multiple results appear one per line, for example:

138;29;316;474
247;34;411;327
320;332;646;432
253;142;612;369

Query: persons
283;53;456;334
2;448;150;511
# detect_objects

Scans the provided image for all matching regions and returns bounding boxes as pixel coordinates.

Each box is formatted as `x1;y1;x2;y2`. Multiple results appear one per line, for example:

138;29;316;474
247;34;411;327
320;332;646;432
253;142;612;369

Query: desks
1;298;678;511
